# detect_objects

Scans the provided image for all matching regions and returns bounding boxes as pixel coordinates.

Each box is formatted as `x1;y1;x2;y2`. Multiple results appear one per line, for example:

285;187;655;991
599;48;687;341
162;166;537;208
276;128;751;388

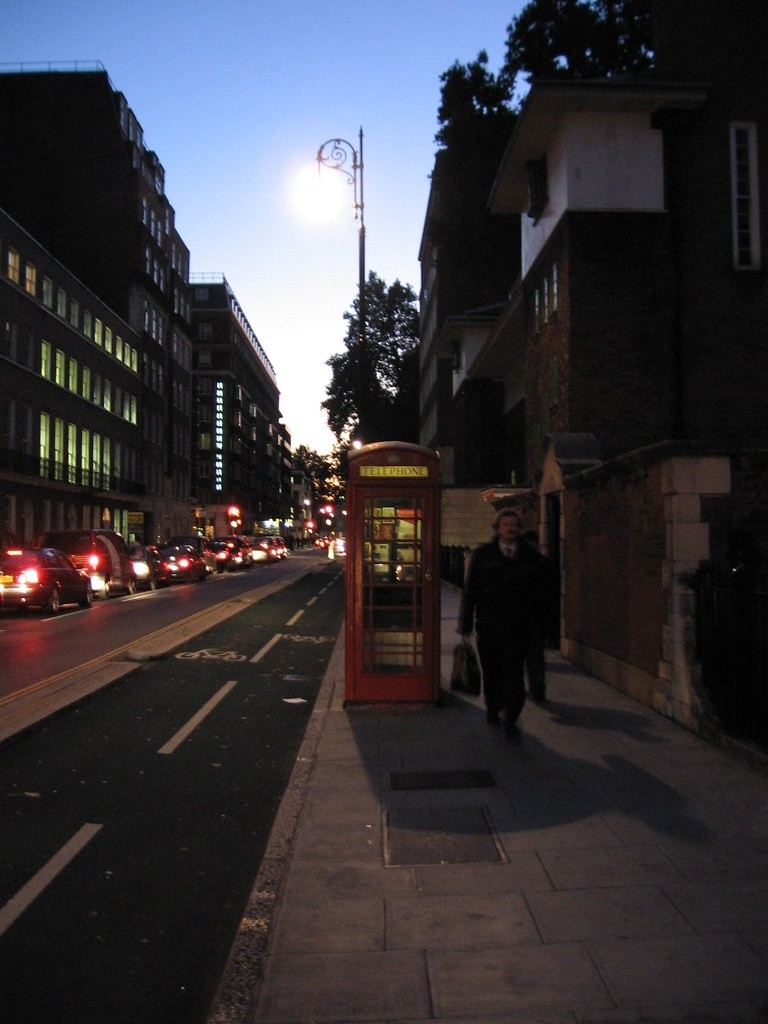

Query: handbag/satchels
450;633;481;696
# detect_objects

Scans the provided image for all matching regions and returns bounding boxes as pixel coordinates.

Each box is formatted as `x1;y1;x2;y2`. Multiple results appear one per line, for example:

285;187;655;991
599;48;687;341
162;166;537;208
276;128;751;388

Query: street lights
304;126;370;444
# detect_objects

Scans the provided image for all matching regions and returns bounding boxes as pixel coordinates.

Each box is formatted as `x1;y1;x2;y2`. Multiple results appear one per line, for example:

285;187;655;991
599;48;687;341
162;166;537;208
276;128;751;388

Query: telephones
362;540;394;585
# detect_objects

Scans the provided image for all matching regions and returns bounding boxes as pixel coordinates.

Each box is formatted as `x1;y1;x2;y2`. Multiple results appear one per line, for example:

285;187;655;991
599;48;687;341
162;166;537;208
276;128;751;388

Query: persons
456;511;552;735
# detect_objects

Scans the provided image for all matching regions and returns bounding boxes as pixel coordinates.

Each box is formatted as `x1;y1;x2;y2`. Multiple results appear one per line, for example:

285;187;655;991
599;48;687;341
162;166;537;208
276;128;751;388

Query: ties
505;547;512;559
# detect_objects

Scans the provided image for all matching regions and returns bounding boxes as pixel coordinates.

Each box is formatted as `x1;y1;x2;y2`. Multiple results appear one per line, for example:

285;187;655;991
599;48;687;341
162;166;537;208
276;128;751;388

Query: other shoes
503;719;521;743
527;690;547;703
487;710;500;726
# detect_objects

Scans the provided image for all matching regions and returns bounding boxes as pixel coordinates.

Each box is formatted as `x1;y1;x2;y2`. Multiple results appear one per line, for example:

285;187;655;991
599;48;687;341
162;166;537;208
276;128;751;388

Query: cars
128;532;289;589
0;545;93;615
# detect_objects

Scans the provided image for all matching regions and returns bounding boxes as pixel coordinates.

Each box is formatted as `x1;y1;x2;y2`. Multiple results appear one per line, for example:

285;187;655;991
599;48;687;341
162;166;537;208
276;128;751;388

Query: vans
42;529;137;599
